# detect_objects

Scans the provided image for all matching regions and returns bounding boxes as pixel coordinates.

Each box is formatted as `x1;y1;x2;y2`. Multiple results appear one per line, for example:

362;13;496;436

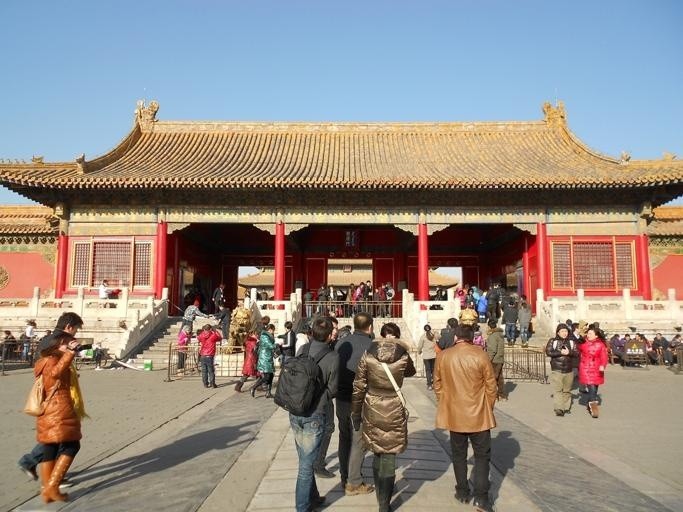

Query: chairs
605;337;681;368
0;336;93;366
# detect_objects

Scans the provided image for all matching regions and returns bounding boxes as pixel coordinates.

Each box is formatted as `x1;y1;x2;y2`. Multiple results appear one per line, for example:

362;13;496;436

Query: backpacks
22;373;64;418
273;343;333;418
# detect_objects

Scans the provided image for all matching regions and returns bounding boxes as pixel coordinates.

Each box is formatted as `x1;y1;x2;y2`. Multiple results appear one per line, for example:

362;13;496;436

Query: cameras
74;344;92;352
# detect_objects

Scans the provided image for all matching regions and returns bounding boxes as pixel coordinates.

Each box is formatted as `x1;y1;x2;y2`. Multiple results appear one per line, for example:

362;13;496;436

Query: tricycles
73;338;108;370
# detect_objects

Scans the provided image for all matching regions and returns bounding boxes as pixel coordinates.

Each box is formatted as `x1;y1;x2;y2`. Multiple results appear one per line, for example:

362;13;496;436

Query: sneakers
58;481;75;488
426;384;433;391
210;381;217;388
18;459;38;481
453;490;468;504
474;497;495;512
297;467;375;511
556;390;599;418
204;382;209;388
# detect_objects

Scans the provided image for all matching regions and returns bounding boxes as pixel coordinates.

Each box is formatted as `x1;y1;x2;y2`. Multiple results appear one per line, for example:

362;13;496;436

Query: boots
40;460;55;502
249;381;263;397
265;383;274;398
377;475;395;512
235;382;245;393
41;454;68;501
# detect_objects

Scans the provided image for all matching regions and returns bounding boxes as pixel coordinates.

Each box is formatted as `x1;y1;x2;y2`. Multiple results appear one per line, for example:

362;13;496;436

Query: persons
246;290;251;299
575;327;608;416
33;333;80;503
304;280;395;317
349;322;416;512
287;318;336;511
196;324;222;387
98;279;108;307
258;288;269;308
235;313;350;397
418;280;530;399
431;327;498;511
545;323;577;417
176;284;228;373
562;318;683;368
17;313;82;488
312;318;339;479
333;314;376;497
1;318;51;363
